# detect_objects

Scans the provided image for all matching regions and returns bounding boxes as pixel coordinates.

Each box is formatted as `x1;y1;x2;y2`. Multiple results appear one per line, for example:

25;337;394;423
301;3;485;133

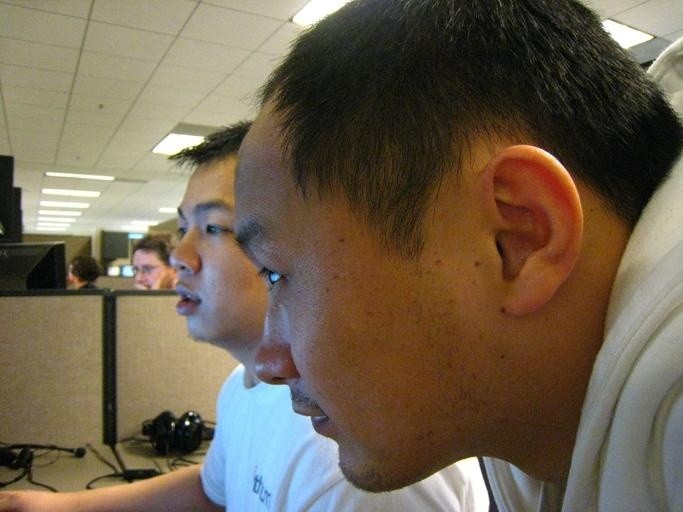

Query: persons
66;254;102;291
0;118;492;512
128;232;174;292
229;2;682;511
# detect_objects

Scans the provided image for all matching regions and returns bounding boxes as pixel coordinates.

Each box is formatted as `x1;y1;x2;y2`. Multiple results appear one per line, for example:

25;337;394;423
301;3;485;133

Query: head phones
0;448;34;487
141;410;215;454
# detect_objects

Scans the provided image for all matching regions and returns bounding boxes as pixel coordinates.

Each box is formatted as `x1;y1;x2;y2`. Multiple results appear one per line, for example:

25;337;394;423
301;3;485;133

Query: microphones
0;442;86;468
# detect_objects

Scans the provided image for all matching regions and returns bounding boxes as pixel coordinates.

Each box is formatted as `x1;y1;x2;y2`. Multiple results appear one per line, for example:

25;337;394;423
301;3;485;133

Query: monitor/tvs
0;243;66;288
121;264;133;276
106;265;120;276
